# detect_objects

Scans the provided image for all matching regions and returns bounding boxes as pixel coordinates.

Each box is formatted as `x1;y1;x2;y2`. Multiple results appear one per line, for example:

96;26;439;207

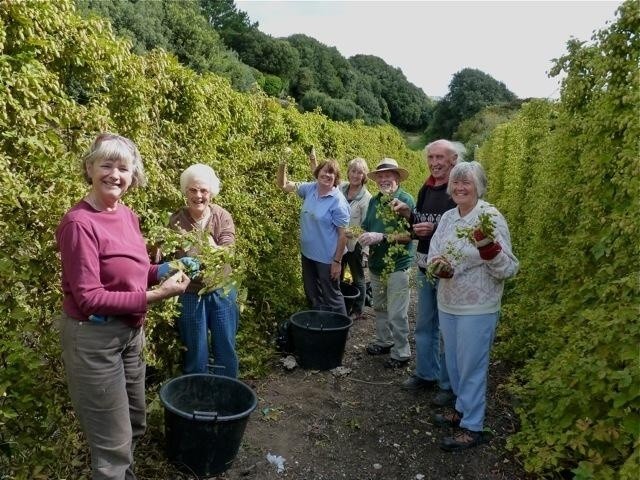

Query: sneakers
441;430;484;450
386;358;409;369
401;375;437;389
367;342;395;355
349;311;362;320
429;411;463;427
433;389;454;407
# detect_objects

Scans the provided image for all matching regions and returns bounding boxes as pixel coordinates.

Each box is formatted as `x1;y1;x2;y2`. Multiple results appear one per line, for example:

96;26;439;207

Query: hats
367;157;409;183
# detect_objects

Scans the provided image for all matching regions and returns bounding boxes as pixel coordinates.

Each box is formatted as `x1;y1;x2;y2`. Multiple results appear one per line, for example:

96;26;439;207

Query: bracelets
381;233;387;244
333;259;341;264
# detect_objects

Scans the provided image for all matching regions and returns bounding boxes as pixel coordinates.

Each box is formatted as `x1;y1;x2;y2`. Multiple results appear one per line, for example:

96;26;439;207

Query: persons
153;163;240;379
423;162;519;450
302;145;372;321
276;148;352;343
343;158;415;368
56;133;200;480
402;140;468;407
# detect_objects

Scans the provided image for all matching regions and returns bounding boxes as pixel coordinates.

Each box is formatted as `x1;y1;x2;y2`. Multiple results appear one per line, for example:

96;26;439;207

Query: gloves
357;231;383;247
158;257;200;286
469;227;493;251
430;257;455;279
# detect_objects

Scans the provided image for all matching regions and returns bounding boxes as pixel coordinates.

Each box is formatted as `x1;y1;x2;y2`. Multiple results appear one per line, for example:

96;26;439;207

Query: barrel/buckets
338;281;361;315
288;304;353;371
156;363;263;477
